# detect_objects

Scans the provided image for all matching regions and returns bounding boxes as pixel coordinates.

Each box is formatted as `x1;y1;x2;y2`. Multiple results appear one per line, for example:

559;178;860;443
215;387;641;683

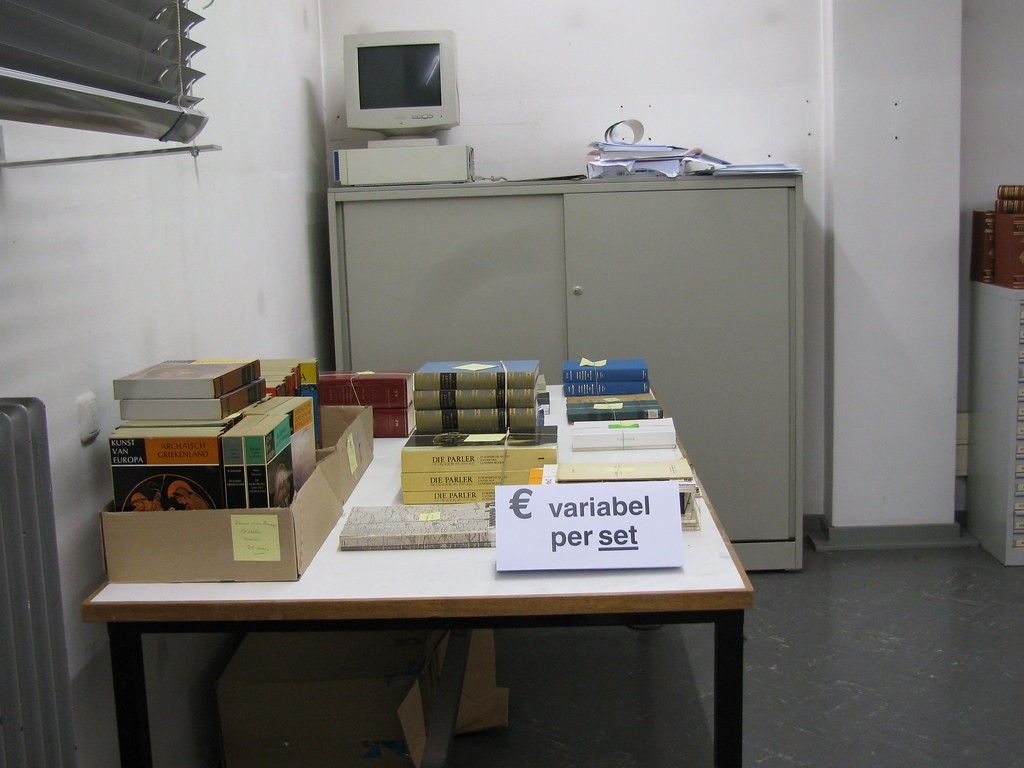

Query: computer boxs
337;144;474;181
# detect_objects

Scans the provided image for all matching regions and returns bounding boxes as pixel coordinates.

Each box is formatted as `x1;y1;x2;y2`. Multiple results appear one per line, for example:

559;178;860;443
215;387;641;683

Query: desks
82;384;755;768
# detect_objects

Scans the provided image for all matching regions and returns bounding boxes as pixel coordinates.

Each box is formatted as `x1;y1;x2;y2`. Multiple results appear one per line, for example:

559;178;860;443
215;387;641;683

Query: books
562;360;701;531
108;358;416;512
338;501;496;552
970;183;1024;289
413;360;551;432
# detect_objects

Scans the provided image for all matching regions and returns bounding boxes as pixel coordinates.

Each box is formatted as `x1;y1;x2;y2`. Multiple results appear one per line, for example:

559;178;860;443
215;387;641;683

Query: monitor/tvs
342;30;460;149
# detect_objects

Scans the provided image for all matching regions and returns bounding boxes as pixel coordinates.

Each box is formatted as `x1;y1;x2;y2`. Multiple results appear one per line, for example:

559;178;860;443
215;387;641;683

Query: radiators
0;397;77;768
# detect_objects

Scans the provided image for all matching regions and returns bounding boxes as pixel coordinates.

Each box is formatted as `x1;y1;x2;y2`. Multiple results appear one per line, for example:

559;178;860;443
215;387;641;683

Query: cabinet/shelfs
966;282;1024;567
328;175;804;569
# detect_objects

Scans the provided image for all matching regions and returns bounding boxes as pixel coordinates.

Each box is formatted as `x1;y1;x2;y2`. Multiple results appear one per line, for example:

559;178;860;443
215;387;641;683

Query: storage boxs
217;627;509;768
102;403;372;587
